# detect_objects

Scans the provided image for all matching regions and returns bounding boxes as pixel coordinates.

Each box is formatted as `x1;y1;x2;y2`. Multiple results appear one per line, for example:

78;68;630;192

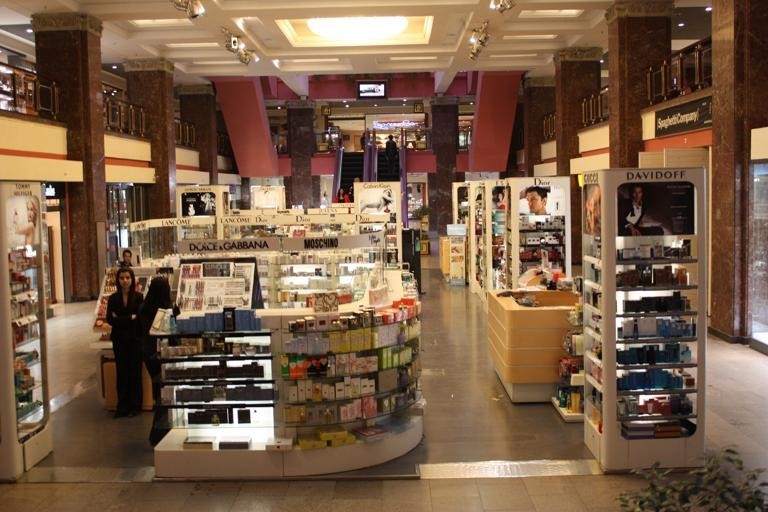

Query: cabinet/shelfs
0;171;53;478
90;173;431;477
438;171;707;478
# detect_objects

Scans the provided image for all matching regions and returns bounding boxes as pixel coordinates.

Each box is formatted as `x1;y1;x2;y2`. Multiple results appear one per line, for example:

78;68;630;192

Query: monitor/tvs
355;79;388;101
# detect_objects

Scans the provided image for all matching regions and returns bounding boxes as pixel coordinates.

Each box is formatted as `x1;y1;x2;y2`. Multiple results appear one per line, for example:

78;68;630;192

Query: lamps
468;0;514;63
169;0;251;67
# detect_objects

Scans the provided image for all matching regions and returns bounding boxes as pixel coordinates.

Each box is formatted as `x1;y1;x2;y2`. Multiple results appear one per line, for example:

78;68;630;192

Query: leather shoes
111;407;141;418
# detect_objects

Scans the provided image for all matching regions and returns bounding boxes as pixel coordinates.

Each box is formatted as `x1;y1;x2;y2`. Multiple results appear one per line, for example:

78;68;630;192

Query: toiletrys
160;252;381;309
554;329;603;433
615;268;698;418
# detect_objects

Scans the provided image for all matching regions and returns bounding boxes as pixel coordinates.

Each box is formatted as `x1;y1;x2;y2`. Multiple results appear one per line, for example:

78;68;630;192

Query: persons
320;191;330;208
12;194;39;245
332;187;351;202
385;134;398;173
497;188;507;209
360;187;393;213
347;176;361;201
619;185;666;239
359;132;365;151
119;249;134;267
520;186;552;215
138;276;181;448
106;266;143;420
